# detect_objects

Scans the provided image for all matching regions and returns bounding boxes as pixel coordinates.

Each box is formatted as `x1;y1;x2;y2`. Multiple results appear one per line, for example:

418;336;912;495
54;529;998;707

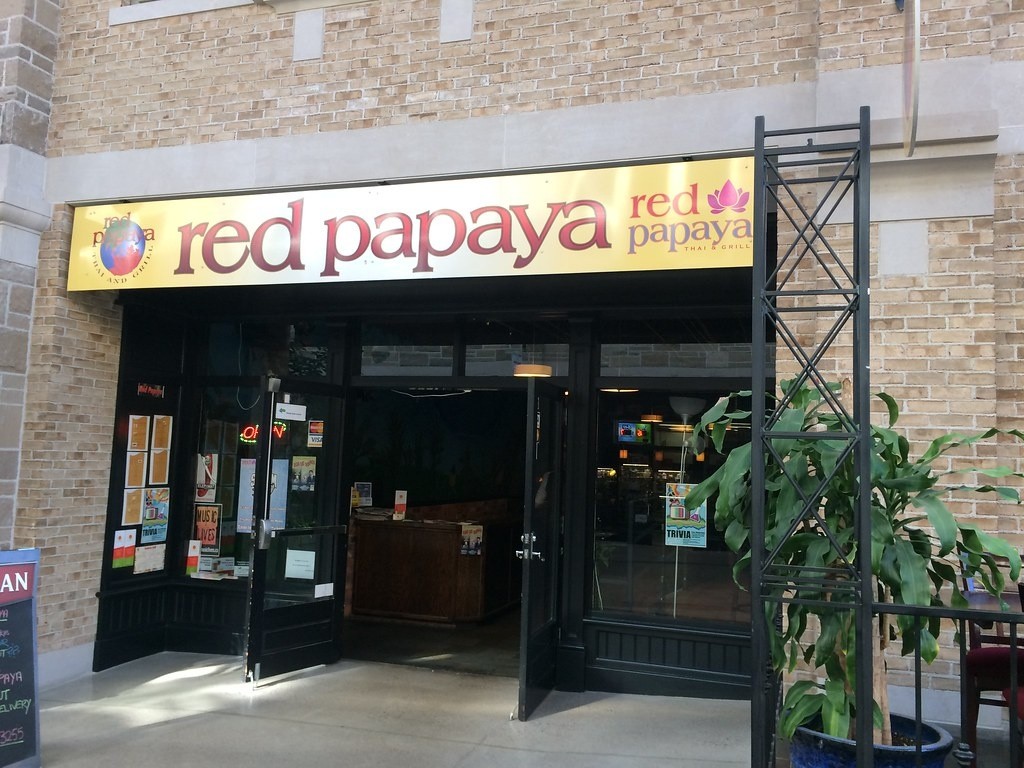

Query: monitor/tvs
611;419;657;447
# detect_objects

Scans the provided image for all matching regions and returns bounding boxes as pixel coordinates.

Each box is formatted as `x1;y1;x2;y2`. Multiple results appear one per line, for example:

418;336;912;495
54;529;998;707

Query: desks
956;590;1023;738
343;498;522;623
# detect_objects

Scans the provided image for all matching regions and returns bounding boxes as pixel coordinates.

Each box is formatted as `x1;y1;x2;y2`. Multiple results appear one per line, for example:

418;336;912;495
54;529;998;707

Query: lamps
641;406;663;423
695;452;705;461
513;324;551;377
620;447;628;459
708;423;731;430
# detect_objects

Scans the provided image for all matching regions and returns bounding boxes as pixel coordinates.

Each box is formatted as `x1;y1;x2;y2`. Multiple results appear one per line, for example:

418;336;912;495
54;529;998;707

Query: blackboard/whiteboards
0;549;42;767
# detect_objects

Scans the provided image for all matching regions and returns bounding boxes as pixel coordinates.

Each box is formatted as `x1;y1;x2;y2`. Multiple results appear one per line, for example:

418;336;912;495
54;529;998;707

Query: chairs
956;545;1023;767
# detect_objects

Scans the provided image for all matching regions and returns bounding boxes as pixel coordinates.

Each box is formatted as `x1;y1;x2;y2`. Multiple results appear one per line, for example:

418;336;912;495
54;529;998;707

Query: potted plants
684;373;1024;768
